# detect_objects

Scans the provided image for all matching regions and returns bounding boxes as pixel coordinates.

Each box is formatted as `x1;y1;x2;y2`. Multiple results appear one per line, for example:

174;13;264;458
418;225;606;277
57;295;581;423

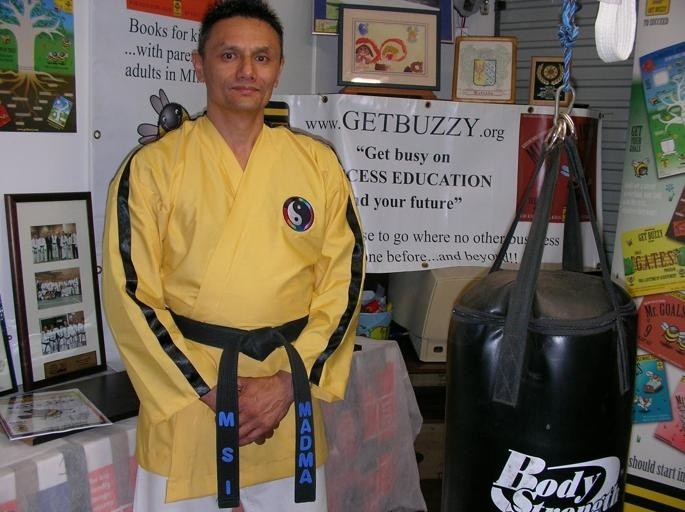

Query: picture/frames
0;188;109;400
335;0;572;110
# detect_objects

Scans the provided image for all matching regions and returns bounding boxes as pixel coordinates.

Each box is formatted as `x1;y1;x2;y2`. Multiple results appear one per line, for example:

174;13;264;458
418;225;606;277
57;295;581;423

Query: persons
32;228;87;356
101;0;369;510
